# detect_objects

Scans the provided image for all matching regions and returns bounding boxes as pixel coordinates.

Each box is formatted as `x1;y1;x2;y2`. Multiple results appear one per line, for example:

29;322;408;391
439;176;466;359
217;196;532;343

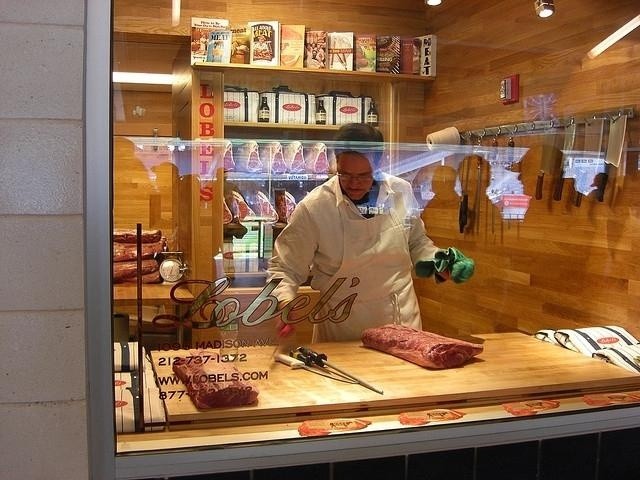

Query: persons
254;34;272;61
265;124;453;342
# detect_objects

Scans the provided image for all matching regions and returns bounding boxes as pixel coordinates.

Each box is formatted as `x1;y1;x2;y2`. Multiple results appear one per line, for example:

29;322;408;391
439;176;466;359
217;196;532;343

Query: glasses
336;171;373;180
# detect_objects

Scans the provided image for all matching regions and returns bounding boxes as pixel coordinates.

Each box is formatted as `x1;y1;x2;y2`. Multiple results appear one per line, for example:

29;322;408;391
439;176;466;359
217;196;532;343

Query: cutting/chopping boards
149;332;640;419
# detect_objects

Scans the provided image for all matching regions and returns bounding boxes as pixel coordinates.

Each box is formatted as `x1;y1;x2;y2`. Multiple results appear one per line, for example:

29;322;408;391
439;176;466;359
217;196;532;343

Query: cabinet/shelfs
191;62;434;349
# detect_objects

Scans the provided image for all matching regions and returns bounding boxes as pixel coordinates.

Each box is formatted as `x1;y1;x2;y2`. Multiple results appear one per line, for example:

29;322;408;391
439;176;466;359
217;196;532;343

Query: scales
159;251;187;286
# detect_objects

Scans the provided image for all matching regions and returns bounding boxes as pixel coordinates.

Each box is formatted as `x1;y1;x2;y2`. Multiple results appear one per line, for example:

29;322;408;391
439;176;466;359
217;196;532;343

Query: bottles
368;100;379;128
258;92;270;122
316;96;326;124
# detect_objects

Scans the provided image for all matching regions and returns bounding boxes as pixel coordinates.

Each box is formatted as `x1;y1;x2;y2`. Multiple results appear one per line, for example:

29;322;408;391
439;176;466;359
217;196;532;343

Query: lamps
533;0;555;18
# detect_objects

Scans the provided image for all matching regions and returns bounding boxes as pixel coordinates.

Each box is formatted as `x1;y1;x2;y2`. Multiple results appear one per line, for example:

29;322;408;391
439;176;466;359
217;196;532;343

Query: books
280;23;305;67
247;20;279;67
305;32;326;68
327;31;354;72
190;17;229;65
207;29;232;63
377;34;401;74
353;35;377;72
411;34;438;77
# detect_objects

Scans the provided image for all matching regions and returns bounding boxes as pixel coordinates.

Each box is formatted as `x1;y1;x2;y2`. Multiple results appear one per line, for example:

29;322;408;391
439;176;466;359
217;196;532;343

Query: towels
415;247;474;283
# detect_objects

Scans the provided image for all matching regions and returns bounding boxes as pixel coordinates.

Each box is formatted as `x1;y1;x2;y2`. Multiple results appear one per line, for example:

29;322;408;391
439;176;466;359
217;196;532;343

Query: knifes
573;117;605;207
534;127;559;200
552;125;576;200
597;115;628;203
272;353;359;384
